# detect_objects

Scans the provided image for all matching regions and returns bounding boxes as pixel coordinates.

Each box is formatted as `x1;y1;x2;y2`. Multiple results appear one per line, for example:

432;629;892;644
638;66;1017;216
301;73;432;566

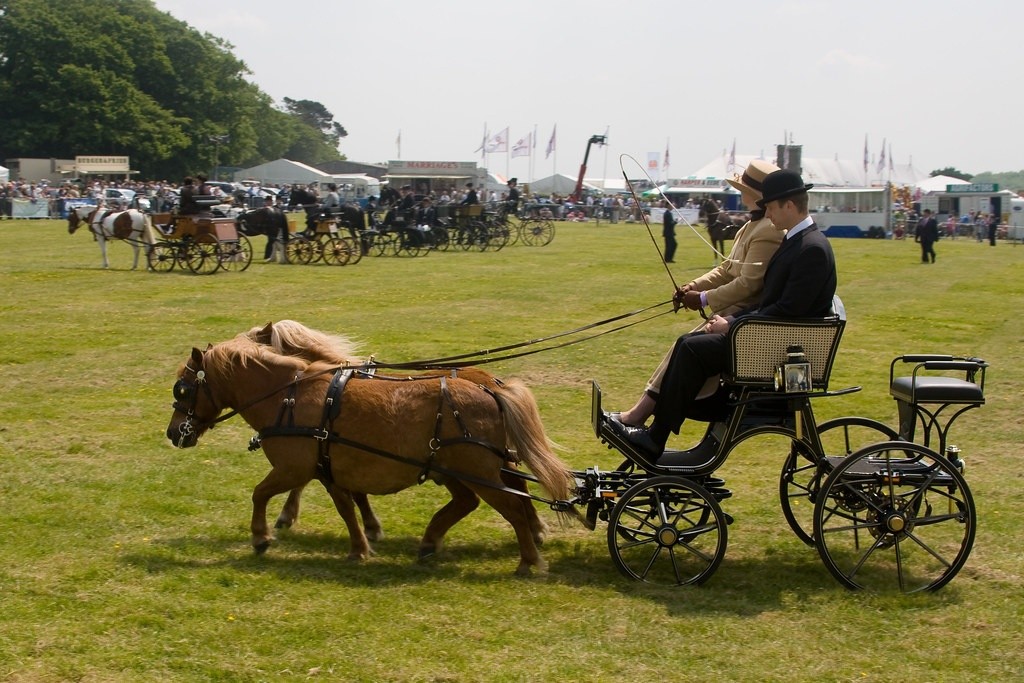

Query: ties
779;233;789;249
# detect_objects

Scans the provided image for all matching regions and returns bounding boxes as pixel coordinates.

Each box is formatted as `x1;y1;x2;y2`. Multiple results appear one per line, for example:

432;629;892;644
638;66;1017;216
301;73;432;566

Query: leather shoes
607;416;664;464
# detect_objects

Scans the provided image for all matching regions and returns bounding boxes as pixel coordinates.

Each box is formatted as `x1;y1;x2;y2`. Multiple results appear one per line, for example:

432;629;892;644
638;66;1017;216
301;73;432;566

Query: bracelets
700;292;707;306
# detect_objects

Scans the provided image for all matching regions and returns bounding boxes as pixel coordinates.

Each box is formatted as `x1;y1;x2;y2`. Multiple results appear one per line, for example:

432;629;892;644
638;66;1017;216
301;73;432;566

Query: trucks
910;184;1024;240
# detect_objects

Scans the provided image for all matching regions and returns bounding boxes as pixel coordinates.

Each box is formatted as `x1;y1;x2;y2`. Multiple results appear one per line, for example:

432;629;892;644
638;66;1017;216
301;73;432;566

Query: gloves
682;290;703;311
673;284;690;313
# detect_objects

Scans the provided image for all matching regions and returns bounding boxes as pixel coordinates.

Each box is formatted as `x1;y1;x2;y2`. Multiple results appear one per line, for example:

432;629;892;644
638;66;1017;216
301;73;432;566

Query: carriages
67;197;253;276
213;205;555;267
165;295;990;595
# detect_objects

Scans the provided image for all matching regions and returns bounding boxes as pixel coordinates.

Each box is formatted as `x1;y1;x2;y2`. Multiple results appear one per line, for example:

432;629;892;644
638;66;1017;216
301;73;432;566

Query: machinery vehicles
560;134;608;217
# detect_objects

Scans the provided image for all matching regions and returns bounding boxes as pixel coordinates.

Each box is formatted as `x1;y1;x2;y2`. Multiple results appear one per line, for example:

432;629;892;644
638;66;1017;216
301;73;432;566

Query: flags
663;147;669;170
476;126;556;159
728;142;735;171
863;139;894;173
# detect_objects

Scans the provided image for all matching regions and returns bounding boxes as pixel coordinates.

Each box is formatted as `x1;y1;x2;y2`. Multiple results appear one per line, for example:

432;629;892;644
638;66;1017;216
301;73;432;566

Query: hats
402;185;412;190
725;158;782;200
755;169;815;208
196;173;209;182
466;182;473;187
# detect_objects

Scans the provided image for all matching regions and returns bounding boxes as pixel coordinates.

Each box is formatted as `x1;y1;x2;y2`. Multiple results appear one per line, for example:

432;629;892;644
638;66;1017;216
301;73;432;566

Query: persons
296;183;339;237
609;170;837;460
378;184;415;228
609;161;789;428
685;198;694;209
197;173;211;195
907;209;938;263
522;192;664;224
161;178;198;233
664;203;679;263
1;175;318;212
946;216;956;237
416;178;519;232
969;208;1008;245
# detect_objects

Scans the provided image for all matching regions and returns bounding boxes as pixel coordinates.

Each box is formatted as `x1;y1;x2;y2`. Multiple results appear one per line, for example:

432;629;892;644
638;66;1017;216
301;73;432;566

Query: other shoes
604;409;640;426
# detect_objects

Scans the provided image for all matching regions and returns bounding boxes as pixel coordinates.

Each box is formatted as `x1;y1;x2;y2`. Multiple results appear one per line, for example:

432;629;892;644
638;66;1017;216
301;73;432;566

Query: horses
168;338;592;577
235;318;549;545
68;205;160;270
379;185;435;209
287;186;370;253
212;206;289;265
698;198;752;261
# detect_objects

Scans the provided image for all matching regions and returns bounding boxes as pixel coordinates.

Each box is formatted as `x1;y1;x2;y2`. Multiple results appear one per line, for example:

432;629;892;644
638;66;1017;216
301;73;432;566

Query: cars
103;188;150;211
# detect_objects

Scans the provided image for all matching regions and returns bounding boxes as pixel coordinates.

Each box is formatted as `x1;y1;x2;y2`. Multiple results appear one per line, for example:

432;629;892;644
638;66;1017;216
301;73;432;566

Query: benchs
889;354;987;404
308;208;344;225
178;195;216;215
730;295;846;391
